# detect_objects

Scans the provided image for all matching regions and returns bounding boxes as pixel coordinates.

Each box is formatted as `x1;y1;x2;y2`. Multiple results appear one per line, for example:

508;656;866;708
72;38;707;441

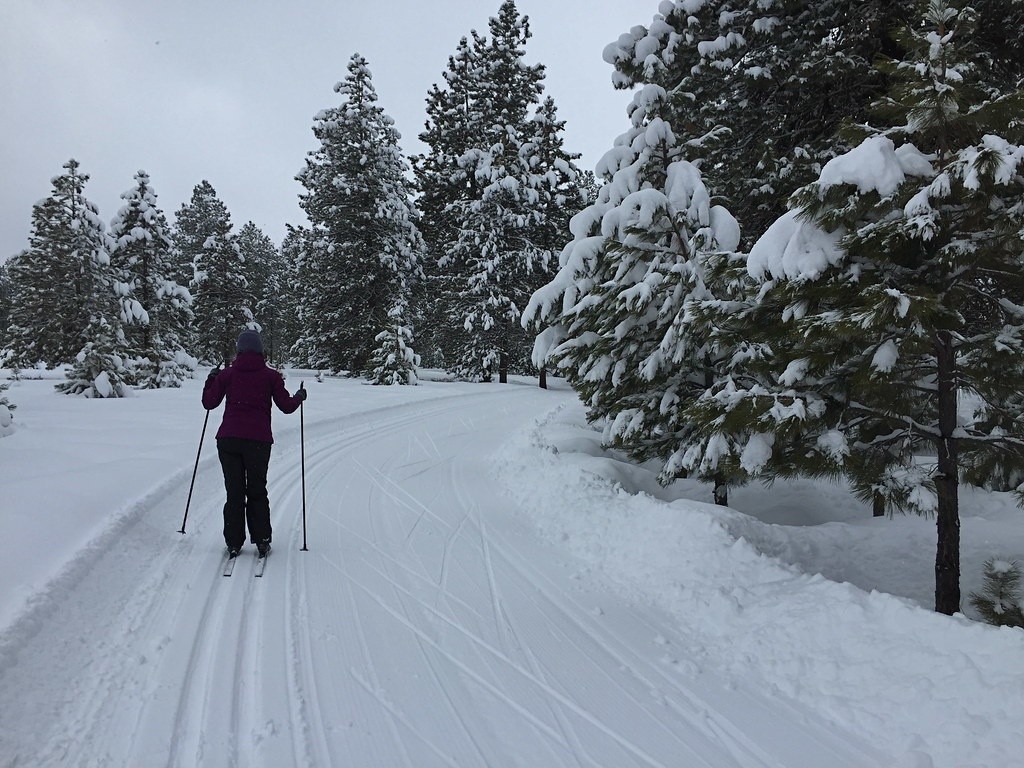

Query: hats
237;330;264;353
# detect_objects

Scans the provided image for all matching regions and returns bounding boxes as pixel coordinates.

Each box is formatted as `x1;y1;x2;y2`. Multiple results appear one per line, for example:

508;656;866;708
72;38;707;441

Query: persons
201;329;307;558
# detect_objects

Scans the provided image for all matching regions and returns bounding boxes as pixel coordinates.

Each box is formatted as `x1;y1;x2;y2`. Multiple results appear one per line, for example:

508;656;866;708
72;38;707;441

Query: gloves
295;389;307;400
210;368;221;378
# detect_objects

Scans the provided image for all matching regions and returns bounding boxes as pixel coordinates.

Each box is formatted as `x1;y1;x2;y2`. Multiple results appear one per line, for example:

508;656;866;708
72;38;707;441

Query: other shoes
256;538;271;558
228;543;241;559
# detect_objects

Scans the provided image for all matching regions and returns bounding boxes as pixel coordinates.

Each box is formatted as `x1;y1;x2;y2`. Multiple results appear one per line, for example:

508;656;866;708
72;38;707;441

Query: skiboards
223;545;269;578
